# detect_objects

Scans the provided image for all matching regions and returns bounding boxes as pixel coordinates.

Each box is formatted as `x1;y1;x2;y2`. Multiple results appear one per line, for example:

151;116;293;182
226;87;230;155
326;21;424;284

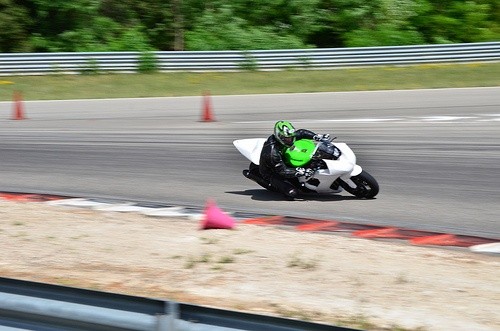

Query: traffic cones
200;89;213;122
11;90;27;121
200;198;236;230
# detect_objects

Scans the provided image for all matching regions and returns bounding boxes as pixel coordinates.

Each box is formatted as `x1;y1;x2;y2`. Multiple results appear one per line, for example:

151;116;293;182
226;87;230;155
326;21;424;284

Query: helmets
274;120;296;148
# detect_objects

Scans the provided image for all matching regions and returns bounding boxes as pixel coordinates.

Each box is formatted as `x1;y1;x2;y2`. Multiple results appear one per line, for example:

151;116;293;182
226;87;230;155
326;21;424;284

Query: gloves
295;168;312;176
313;134;331;141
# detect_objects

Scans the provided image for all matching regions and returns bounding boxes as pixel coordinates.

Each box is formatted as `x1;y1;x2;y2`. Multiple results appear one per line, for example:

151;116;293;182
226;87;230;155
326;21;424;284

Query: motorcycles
233;133;379;203
